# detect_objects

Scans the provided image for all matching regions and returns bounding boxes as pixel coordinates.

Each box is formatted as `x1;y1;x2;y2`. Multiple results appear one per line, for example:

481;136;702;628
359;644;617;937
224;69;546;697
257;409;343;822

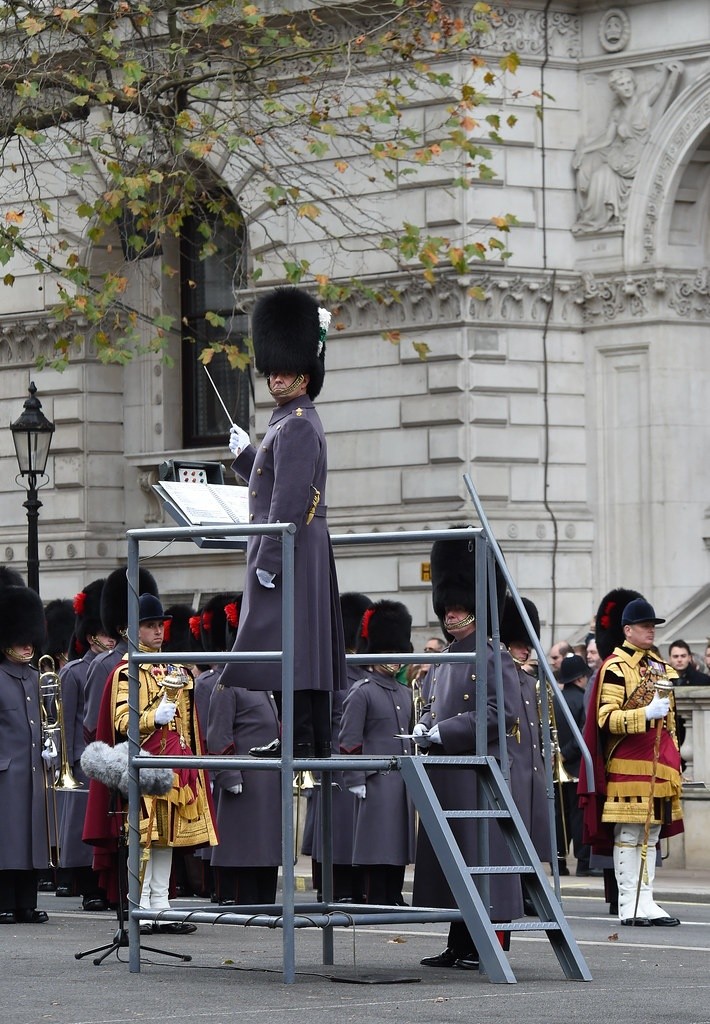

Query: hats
0;562;418;665
622;598;666;626
502;596;540;648
553;655;591;684
251;287;332;402
430;525;506;642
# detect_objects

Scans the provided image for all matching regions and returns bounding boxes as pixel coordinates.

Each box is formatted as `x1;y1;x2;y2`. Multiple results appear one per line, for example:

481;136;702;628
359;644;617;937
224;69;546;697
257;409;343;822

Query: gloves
411;723;433;747
256;567;276;589
347;785;367;799
41;738;58;761
227;783;244;795
643;690;671;720
229;423;251;455
425;723;445;745
154;692;177;724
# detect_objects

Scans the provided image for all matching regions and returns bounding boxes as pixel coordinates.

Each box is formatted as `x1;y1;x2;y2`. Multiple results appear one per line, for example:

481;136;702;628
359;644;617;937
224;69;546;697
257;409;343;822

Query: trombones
38;653;84;871
293;769;321;865
409;676;427;839
535;678;576;859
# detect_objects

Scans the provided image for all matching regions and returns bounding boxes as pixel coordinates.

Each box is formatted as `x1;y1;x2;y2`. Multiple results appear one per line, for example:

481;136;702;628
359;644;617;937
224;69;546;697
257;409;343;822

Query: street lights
8;381;57;589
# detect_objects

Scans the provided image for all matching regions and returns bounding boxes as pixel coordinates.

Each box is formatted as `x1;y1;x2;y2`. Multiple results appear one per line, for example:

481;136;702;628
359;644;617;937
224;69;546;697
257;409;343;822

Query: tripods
74;795;192;966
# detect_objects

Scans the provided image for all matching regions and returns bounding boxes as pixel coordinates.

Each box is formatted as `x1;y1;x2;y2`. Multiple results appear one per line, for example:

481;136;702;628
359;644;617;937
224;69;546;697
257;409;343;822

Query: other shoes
575;860;604;878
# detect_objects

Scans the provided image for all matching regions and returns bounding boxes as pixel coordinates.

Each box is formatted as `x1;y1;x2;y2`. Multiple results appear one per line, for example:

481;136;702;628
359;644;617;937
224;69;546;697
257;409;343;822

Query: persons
0;566;710;926
217;285;348;761
411;522;522;968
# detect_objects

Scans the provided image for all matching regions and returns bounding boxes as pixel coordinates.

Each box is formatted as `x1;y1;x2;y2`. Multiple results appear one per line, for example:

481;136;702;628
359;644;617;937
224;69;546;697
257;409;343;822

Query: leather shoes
419;949;467;967
621;915;649;926
155;923;197;934
651;915;682;927
456;954;483;970
248;734;314;758
139;923;154;935
1;877;129;923
310;739;331;758
